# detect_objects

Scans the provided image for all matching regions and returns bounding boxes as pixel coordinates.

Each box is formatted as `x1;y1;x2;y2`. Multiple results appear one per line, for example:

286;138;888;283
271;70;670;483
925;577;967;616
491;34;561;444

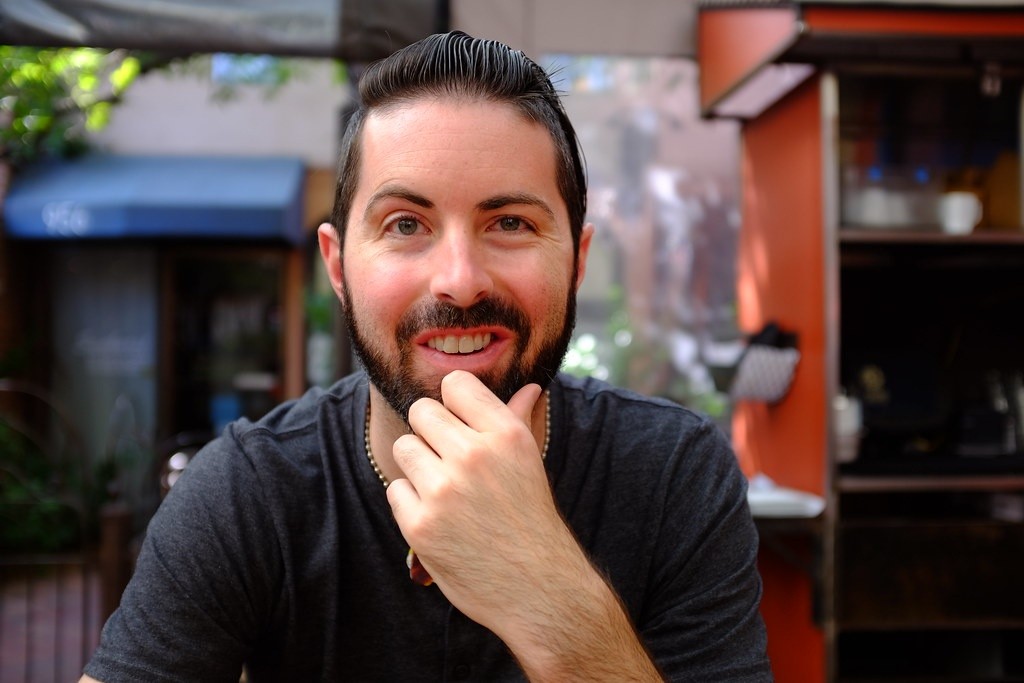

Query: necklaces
365;388;551;588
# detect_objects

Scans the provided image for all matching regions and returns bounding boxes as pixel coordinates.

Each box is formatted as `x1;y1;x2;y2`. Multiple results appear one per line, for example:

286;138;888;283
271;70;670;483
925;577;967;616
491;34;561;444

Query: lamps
701;324;824;521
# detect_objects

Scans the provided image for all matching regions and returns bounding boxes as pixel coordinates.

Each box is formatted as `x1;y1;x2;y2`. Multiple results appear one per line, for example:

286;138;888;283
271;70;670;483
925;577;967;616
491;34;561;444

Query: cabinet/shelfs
698;0;1024;683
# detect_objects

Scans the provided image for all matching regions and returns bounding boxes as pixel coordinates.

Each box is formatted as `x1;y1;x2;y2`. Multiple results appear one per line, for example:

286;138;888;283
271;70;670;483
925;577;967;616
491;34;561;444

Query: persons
73;29;775;683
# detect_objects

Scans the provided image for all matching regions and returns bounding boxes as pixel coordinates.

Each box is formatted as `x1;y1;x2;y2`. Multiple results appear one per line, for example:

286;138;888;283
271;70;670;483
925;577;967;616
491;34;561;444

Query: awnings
3;155;306;243
